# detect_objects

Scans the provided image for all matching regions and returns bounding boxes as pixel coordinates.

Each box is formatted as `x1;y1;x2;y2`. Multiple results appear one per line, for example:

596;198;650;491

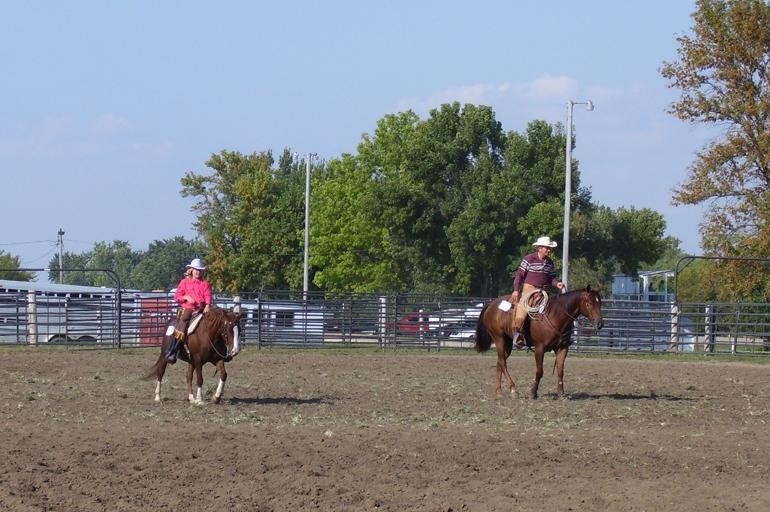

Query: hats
185;259;207;270
532;237;557;249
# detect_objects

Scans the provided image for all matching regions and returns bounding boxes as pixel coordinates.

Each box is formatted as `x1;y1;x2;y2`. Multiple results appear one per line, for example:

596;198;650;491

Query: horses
137;307;245;411
472;283;604;403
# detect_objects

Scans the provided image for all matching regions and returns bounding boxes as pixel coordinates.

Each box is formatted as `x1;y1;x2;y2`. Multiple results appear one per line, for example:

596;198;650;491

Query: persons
162;257;215;365
510;234;568;347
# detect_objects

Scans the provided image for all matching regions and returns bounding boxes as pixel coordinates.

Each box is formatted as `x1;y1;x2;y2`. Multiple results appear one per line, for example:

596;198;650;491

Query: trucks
0;280;327;348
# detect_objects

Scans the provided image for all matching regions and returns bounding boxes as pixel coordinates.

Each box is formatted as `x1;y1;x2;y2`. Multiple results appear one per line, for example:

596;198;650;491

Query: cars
371;306;479;343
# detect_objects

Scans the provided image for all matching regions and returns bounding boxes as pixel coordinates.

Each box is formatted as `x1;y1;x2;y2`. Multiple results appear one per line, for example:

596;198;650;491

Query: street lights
559;97;595;297
302;151;318;300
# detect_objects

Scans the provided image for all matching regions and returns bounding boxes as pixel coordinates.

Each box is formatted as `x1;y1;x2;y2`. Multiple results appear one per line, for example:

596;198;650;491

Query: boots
169;331;185;362
513;328;523;350
